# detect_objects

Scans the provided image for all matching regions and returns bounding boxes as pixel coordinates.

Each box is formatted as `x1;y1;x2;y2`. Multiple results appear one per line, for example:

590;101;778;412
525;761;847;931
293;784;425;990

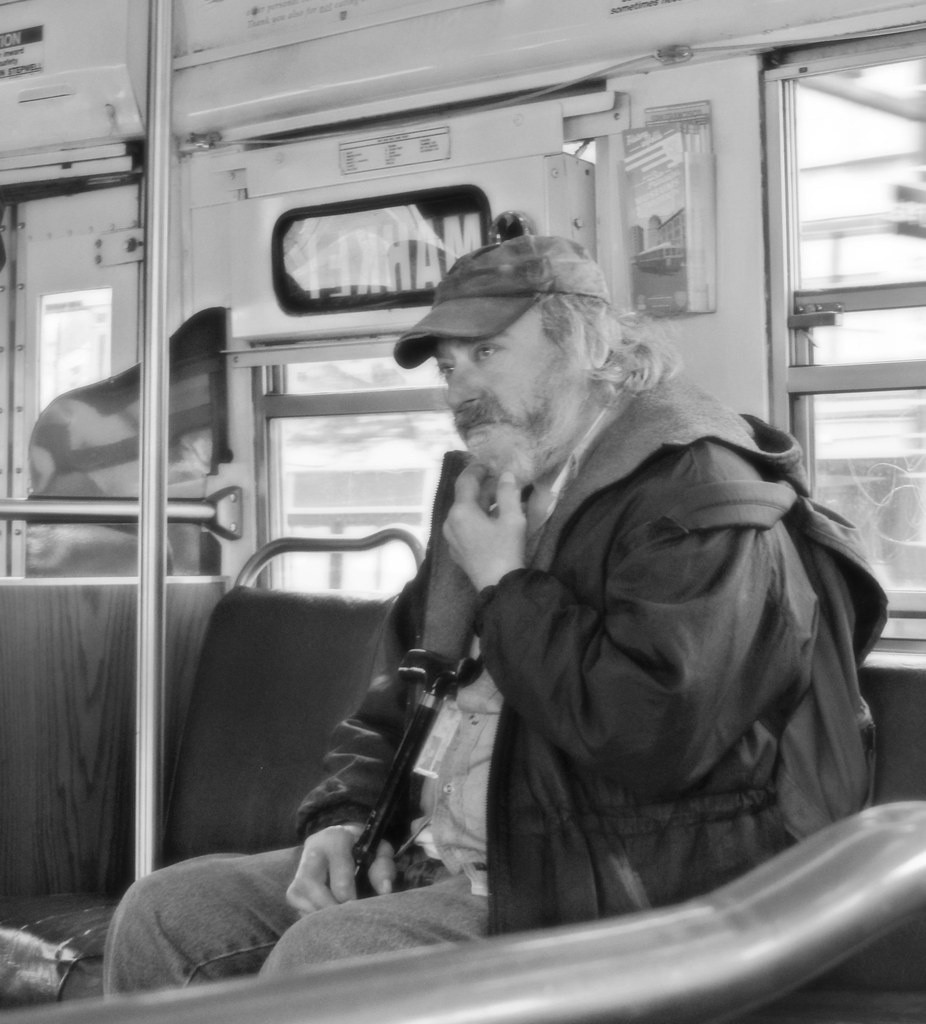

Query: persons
103;234;890;996
23;305;228;578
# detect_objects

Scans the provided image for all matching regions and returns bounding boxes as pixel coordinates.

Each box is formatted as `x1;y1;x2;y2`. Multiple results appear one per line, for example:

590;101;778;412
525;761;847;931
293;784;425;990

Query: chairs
0;528;426;1010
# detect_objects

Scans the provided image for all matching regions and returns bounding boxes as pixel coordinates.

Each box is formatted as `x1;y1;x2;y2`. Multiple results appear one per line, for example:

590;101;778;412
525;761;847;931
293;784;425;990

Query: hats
394;235;612;368
